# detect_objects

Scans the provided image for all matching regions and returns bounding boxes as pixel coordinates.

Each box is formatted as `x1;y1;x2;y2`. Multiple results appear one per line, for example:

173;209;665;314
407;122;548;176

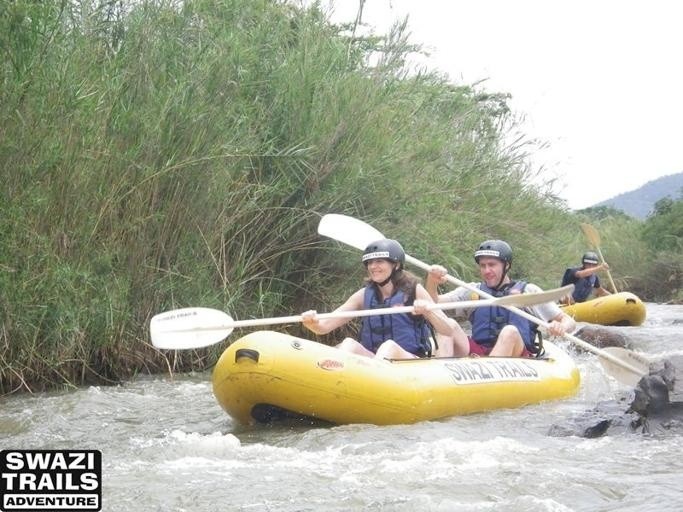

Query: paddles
579;223;619;294
317;213;646;377
150;283;578;350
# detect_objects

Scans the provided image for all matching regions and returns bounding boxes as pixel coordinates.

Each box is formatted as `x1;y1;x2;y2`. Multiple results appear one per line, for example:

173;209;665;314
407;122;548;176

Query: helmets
361;240;405;272
474;240;512;273
582;252;598;265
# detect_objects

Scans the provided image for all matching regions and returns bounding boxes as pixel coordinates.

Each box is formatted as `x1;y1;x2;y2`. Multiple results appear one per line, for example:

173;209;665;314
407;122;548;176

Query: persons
425;239;574;359
558;253;613;305
301;238;454;362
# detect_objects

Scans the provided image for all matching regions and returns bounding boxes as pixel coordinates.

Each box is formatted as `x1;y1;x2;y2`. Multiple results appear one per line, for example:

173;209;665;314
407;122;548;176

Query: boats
558;290;647;326
211;330;581;430
